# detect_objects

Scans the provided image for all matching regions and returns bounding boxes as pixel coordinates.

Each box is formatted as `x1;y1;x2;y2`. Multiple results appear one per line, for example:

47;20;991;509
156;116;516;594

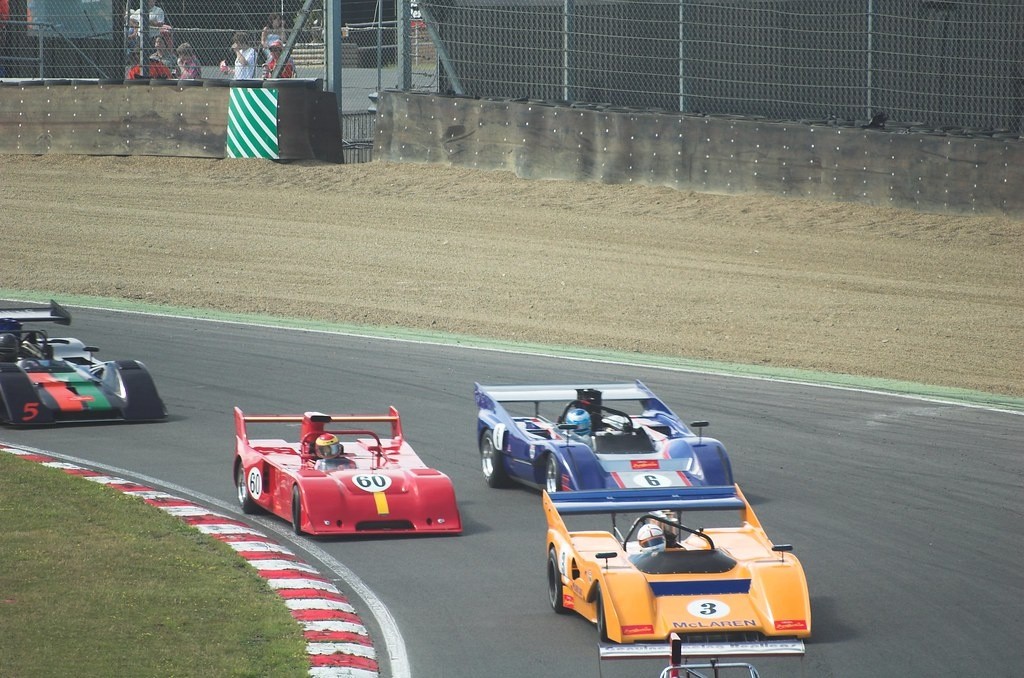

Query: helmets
0;333;19;362
566;409;592;436
637;524;666;553
315;433;341;460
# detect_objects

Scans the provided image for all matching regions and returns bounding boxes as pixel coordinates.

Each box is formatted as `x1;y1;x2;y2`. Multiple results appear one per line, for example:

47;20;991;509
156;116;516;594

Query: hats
269;41;282;51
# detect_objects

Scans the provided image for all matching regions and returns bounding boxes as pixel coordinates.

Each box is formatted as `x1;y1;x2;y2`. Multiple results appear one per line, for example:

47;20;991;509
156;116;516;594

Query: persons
176;43;201;79
314;433;356;472
220;58;230;75
126;0;180;79
230;32;259;79
261;11;287;61
265;40;296;78
637;524;666;553
0;333;19;362
565;409;592;448
